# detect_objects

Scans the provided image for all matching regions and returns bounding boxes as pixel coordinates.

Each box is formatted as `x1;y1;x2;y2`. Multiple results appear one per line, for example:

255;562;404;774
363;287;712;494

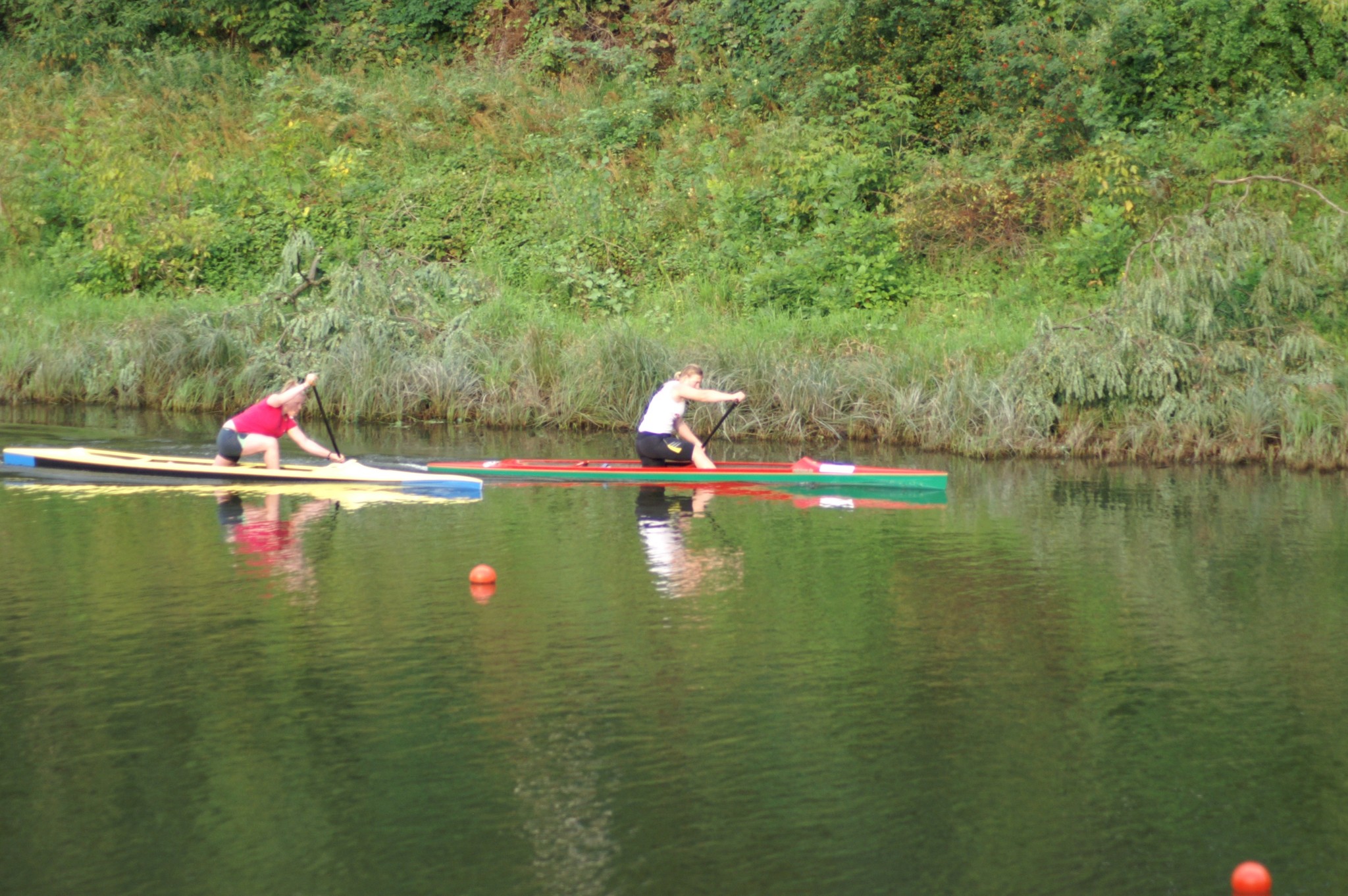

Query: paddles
313;385;340;458
701;398;738;448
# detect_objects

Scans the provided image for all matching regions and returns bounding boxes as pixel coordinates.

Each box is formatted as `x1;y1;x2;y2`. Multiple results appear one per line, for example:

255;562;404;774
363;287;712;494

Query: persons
636;364;745;470
212;374;345;470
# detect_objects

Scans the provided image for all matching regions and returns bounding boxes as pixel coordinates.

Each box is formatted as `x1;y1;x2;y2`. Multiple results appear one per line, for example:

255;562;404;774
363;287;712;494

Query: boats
3;440;483;489
278;457;948;489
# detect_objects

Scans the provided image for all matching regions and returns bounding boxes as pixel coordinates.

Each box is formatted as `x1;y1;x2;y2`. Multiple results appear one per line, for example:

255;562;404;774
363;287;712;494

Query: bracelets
327;453;332;460
